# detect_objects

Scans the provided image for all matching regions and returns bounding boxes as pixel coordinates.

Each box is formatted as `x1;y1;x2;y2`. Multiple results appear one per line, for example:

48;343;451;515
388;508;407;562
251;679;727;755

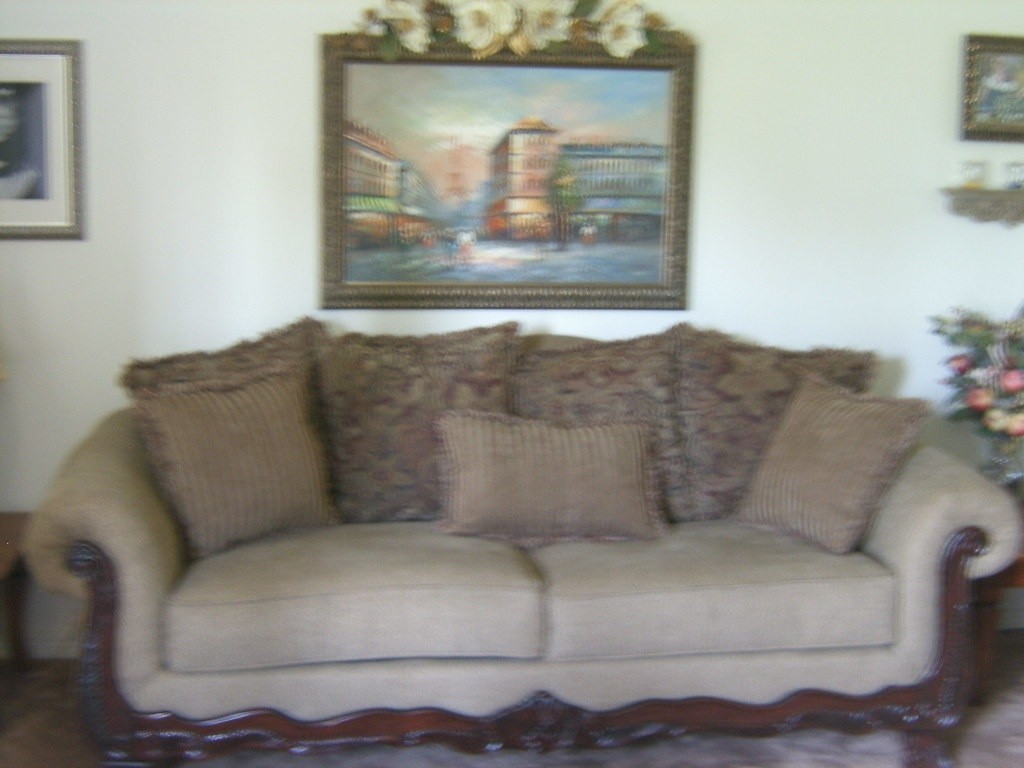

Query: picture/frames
319;0;697;313
962;34;1024;142
0;39;84;239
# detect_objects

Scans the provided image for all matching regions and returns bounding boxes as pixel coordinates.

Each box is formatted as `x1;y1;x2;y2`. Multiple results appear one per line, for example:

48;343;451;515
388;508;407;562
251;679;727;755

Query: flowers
935;312;1024;456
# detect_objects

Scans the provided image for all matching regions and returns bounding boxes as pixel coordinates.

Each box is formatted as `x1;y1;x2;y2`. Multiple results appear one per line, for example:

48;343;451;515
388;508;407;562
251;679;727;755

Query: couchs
20;410;1023;768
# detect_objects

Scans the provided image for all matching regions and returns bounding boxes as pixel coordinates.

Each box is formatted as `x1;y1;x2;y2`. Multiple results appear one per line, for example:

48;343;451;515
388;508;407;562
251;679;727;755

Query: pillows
118;315;932;554
